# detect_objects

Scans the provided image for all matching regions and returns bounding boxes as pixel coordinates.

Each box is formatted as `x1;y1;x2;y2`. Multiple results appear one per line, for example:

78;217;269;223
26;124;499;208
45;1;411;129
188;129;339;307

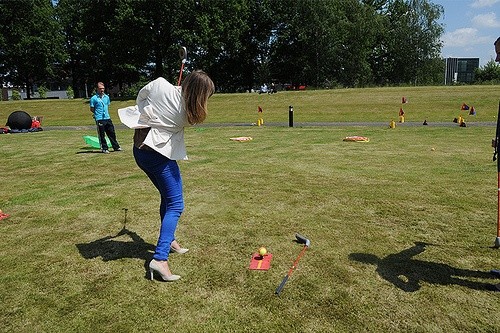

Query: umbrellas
83;134;118;149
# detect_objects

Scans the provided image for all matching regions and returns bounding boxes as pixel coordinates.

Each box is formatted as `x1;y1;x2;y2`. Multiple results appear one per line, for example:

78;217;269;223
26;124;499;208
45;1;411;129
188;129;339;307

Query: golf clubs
178;46;187;86
275;232;311;294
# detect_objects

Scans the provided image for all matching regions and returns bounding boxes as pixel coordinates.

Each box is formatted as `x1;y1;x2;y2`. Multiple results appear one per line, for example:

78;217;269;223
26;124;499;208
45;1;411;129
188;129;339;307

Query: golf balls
257;246;267;256
431;147;435;151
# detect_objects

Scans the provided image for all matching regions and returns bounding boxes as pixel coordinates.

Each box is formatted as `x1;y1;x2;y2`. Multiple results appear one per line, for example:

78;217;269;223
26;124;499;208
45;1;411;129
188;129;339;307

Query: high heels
171;246;189;255
149;259;181;281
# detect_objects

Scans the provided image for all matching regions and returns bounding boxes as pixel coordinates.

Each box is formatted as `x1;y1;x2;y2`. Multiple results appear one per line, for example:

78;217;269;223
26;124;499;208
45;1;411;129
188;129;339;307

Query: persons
259;83;267;94
90;82;122;154
461;102;470;110
453;114;464;124
133;69;215;281
255;104;266;127
492;36;500;159
268;82;277;94
469;106;477;116
422;119;428;125
388;120;397;128
401;96;407;104
399;108;406;122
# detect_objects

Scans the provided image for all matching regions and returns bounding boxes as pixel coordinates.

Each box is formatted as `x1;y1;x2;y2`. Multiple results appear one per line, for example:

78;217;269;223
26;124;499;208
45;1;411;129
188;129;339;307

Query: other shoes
113;147;122;151
102;149;111;154
